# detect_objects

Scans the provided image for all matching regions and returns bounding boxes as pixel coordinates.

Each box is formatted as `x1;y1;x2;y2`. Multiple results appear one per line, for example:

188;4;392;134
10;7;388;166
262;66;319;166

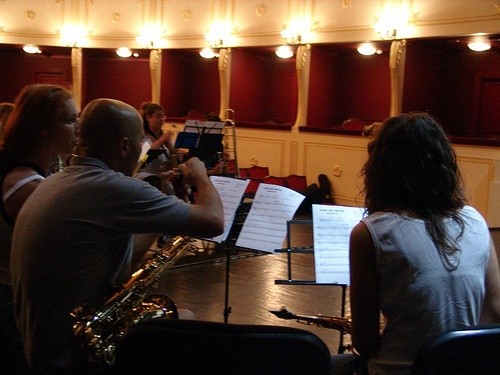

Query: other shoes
158;236;170;248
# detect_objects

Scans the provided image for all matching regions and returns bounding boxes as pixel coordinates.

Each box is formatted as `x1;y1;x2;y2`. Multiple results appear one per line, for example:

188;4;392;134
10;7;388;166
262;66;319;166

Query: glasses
150;114;166;120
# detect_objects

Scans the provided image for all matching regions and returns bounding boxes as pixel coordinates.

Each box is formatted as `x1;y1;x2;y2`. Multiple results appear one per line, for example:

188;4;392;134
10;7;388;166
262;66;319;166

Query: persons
331;113;500;375
202;115;229;177
10;98;224;375
0;85;84;375
136;102;194;247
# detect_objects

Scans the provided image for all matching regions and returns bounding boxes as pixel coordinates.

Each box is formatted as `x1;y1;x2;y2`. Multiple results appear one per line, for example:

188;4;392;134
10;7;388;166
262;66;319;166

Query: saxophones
68;143;195;370
268;305;386;335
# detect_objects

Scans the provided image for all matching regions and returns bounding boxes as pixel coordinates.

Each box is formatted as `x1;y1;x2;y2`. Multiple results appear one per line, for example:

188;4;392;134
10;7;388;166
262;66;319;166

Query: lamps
274;46;294;58
356;43;378;55
198;48;220;59
22;44;42;54
115;47;140;58
464;34;493;52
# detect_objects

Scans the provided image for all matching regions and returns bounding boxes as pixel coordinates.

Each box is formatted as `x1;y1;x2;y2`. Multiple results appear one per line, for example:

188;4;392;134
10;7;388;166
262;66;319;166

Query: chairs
408;325;500;375
226;159;307;197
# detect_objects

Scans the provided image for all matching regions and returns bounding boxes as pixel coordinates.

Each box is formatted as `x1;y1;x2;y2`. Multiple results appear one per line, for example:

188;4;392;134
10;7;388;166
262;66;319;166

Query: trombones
219;108;239;178
